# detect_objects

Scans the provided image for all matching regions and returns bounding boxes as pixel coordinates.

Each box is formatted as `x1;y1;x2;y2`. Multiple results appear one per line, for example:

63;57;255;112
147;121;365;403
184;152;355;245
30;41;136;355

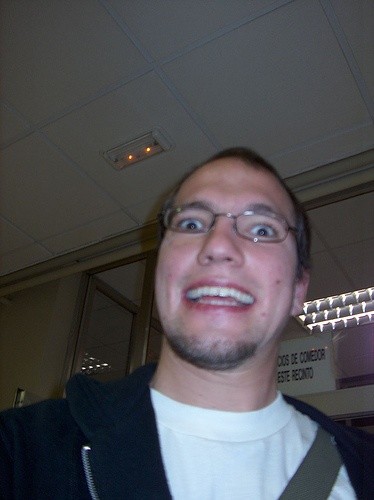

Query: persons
0;146;374;499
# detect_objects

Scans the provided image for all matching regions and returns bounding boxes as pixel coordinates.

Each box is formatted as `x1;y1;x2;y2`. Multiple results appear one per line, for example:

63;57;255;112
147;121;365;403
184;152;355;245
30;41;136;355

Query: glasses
157;204;302;244
